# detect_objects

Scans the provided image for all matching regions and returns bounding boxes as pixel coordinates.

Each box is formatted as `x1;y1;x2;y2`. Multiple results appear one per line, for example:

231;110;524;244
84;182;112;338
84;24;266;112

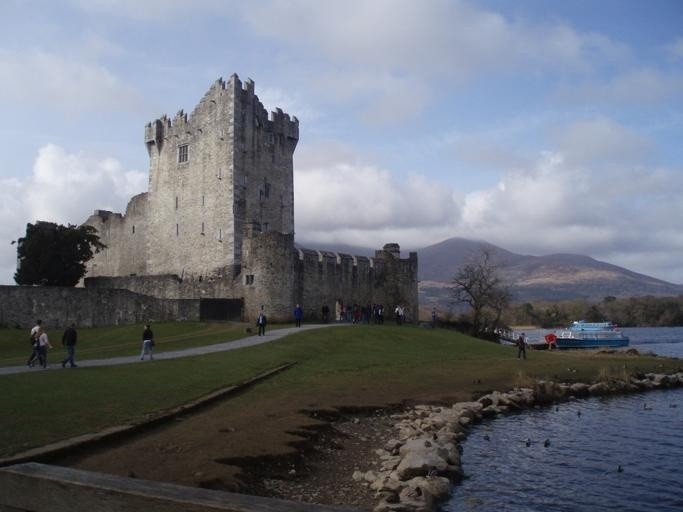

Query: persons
431;307;436;328
27;325;53;368
256;312;267;336
322;302;330;321
61;322;78;368
293;303;303;327
26;319;44;365
340;302;384;324
140;324;155;360
395;304;405;325
515;332;528;360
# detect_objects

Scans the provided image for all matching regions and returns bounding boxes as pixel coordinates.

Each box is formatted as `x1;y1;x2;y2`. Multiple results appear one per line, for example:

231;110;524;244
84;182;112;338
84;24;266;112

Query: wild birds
526;438;531;446
643;402;653;410
556;407;559;412
616;465;624;472
484;432;490;440
544;438;550;447
576;408;581;415
668;400;679;408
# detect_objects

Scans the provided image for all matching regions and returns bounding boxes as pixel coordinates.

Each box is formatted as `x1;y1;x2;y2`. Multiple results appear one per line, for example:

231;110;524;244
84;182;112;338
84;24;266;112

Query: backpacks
30;332;37;345
32;338;40;350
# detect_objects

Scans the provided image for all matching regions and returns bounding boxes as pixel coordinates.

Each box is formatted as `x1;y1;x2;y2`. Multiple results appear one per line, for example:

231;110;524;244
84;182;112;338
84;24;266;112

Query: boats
554;319;629;349
